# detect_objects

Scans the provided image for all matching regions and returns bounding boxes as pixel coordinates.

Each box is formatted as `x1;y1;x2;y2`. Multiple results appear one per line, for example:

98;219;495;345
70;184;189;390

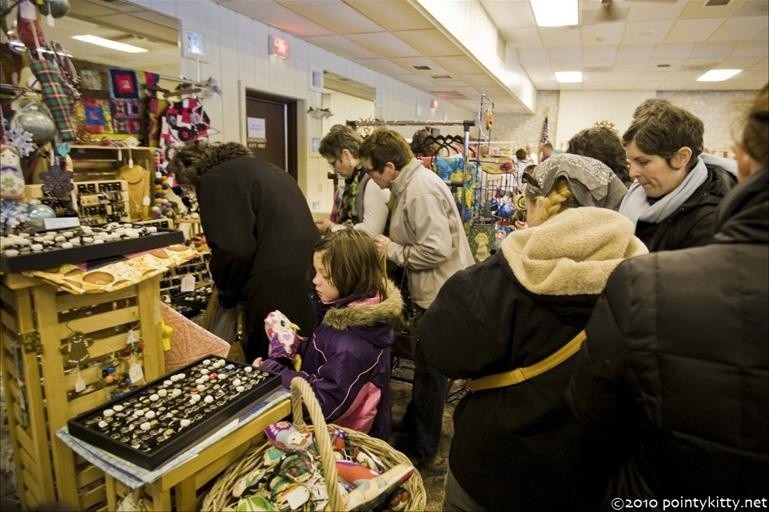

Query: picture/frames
71;58;139;133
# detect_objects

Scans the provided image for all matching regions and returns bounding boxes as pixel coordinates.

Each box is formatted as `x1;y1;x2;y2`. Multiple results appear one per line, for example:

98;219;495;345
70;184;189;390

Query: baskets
195;372;430;512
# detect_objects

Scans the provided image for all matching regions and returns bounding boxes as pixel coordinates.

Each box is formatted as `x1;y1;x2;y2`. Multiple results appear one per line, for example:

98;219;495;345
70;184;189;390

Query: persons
167;138;321;365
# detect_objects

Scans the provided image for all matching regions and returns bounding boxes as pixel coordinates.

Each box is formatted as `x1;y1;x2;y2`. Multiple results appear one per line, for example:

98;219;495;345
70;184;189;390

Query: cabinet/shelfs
0;272;294;511
